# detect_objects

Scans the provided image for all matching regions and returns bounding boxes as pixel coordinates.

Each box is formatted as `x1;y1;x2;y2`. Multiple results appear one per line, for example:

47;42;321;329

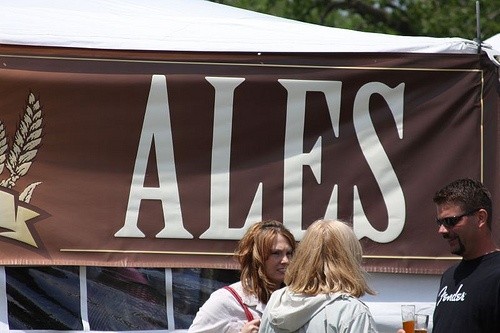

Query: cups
401;305;415;333
414;314;429;333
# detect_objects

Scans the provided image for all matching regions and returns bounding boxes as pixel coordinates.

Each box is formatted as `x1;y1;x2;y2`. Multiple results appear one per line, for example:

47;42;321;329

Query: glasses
436;208;477;228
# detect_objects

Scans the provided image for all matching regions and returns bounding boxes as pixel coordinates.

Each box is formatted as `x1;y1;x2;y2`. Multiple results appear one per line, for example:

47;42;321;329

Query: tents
0;0;500;333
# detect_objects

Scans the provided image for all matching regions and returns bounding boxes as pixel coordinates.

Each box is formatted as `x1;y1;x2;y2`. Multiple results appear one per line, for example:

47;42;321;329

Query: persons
397;177;500;333
258;219;380;333
188;220;296;333
